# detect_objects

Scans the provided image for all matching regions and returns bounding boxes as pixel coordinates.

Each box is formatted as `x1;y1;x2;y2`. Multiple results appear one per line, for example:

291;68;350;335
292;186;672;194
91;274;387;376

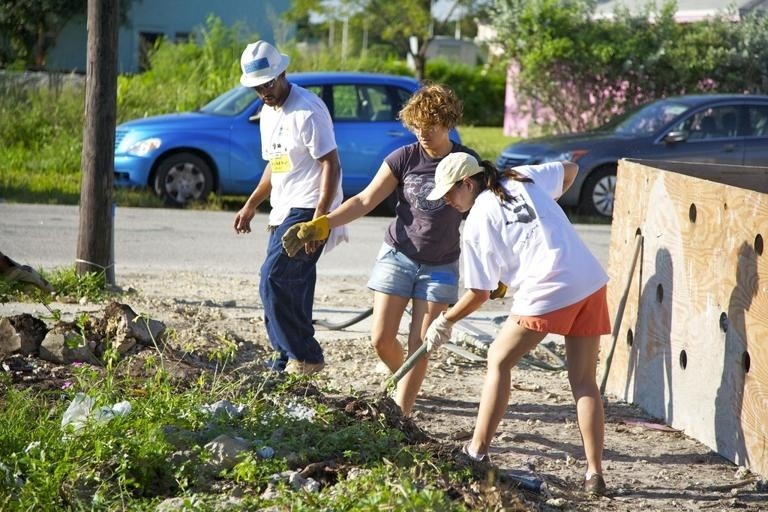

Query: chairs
688;112;737;142
371;94;392;122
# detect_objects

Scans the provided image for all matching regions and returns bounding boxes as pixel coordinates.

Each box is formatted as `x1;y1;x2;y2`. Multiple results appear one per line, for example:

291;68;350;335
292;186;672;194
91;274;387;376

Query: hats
425;151;485;201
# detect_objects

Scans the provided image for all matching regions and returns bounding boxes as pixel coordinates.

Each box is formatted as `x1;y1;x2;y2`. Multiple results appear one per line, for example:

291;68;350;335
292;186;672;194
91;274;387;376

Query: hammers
599;237;643;408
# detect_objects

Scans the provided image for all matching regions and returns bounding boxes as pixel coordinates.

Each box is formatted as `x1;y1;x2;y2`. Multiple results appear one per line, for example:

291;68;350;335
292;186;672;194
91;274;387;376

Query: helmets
239;39;290;88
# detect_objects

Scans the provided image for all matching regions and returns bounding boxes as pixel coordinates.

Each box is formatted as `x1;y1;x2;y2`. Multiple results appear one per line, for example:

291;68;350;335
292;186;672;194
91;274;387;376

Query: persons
425;151;611;494
233;40;350;380
282;82;484;417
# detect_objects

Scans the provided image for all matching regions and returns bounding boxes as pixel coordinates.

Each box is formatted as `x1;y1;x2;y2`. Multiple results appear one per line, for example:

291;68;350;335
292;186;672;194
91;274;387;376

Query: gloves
424;310;457;353
489;281;508;300
280;215;330;259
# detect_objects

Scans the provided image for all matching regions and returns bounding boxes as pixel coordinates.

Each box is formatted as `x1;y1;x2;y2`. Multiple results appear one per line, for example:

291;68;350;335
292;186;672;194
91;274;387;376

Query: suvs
106;71;463;209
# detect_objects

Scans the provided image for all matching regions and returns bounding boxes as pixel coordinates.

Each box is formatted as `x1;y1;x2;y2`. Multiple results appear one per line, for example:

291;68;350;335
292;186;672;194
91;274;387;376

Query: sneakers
283;355;327;377
581;472;607;498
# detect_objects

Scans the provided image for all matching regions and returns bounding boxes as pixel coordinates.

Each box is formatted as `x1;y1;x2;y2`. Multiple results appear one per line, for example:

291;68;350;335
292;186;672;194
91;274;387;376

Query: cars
493;91;768;221
